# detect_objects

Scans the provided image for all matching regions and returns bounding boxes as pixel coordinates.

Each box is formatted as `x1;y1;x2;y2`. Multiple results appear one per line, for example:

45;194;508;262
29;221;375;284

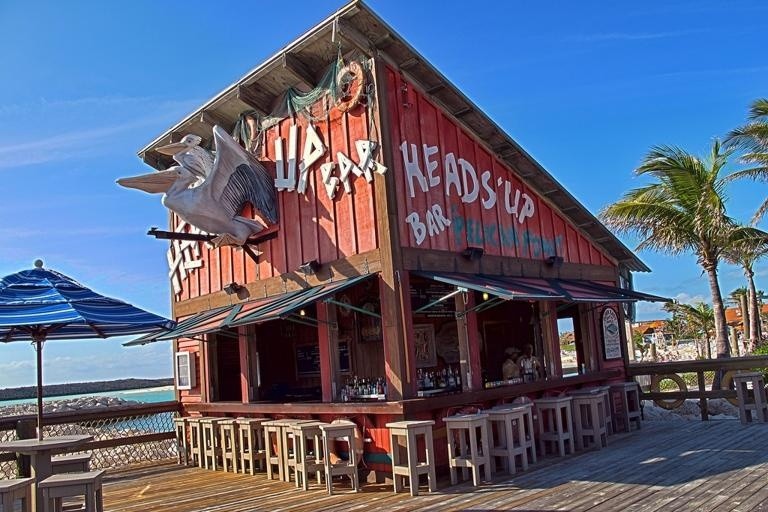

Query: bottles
416;365;460;391
485;378;523;388
337;376;386;400
579;363;586;374
520;358;536;383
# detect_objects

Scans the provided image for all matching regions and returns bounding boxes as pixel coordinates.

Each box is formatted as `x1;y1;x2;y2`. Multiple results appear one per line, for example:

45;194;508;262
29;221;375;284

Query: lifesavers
721;369;753;406
651;373;687;409
453;397;540;454
272;418;364;466
332;60;367;112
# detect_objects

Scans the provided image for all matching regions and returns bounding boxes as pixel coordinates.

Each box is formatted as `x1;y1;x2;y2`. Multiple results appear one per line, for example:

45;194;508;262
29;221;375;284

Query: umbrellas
0;259;177;442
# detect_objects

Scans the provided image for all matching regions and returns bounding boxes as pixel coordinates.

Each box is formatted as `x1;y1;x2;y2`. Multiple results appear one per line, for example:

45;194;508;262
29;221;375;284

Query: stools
162;412;365;497
382;375;643;499
730;371;766;425
0;475;38;512
49;452;95;474
36;469;108;512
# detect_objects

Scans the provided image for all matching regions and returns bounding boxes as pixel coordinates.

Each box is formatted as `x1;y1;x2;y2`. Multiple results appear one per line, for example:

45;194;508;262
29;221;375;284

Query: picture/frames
413;322;439;370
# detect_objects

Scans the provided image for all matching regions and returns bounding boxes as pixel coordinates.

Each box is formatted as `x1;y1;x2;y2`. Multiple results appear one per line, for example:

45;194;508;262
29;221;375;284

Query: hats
505;347;522;354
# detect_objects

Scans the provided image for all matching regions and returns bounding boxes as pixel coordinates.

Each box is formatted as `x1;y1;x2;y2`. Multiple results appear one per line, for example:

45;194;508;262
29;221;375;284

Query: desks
0;433;94;512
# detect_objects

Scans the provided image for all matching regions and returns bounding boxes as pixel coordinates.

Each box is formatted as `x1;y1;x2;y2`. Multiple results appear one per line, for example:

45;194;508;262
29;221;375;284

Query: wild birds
112;124;279;252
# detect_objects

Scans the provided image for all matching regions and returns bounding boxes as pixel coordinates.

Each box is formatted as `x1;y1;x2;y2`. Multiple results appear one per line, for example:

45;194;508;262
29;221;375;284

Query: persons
502;348;521;382
517;344;541;382
